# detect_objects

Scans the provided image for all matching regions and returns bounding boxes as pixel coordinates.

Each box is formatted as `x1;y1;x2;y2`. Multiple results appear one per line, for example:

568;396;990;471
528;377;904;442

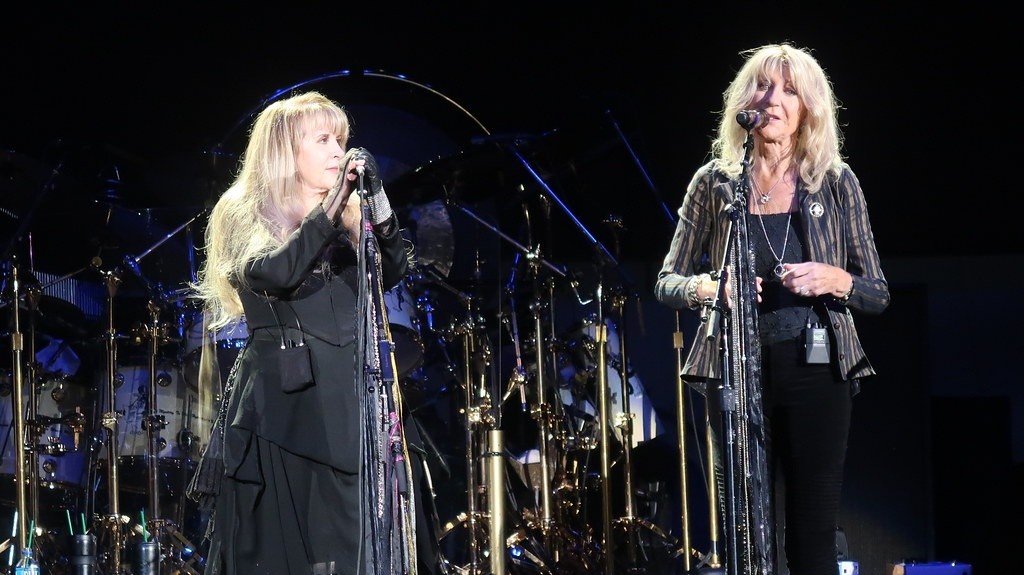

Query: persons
188;89;448;575
656;43;892;574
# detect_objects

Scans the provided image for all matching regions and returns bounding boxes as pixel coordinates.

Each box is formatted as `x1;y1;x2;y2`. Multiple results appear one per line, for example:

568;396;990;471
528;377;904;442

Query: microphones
737;108;771;129
355;164;366;177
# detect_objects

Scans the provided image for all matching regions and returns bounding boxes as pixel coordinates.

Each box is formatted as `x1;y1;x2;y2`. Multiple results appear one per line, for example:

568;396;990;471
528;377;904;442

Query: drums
523;314;665;479
95;358;220;478
1;372;93;493
382;277;425;381
182;294;250;397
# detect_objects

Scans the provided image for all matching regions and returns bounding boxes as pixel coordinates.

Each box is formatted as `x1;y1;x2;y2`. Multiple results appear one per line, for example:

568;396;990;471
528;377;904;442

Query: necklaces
744;158;801;277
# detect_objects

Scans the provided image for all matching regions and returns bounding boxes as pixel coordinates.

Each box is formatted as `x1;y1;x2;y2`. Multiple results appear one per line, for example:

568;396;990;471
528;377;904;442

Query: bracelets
688;274;706;306
844;272;854;301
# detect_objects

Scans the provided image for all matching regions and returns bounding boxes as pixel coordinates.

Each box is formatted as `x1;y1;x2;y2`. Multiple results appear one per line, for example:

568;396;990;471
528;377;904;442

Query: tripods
0;186;776;574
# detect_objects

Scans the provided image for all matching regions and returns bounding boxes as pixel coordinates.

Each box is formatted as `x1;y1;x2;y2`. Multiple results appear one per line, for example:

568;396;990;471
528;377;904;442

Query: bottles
72;532;96;575
14;547;40;575
137;540;160;575
